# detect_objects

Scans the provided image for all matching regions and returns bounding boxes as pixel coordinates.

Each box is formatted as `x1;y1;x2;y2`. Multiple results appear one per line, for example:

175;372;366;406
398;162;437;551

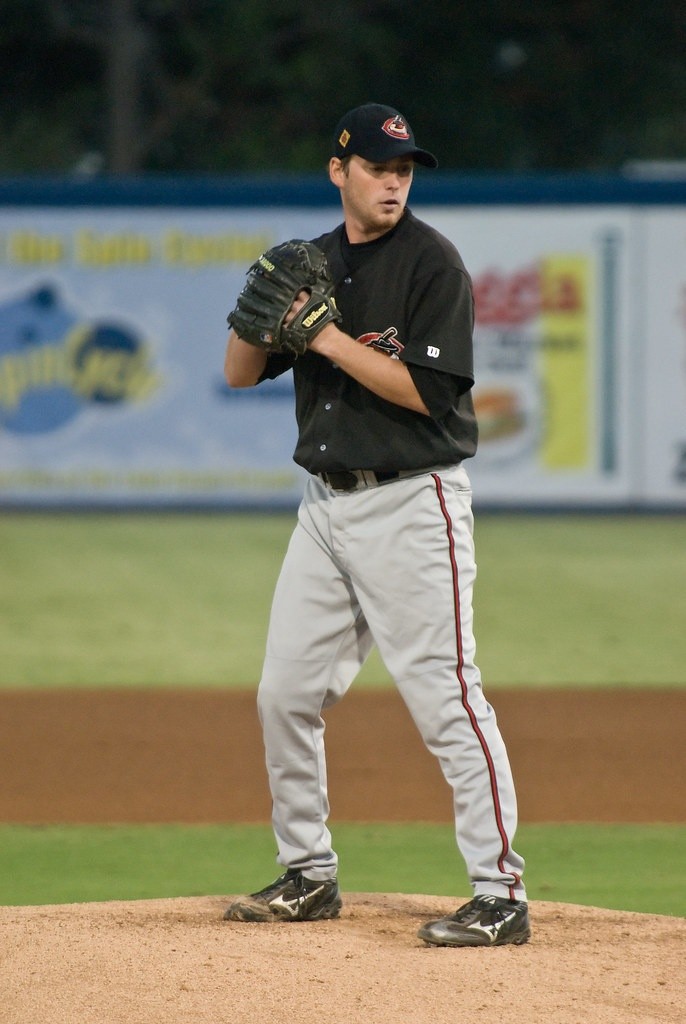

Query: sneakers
417;893;530;948
224;868;341;923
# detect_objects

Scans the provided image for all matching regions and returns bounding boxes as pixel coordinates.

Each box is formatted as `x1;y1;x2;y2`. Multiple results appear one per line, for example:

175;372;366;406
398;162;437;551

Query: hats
334;103;439;170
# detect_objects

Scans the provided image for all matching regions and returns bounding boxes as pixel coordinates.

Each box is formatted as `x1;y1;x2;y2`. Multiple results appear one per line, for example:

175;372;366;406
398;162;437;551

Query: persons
223;104;532;947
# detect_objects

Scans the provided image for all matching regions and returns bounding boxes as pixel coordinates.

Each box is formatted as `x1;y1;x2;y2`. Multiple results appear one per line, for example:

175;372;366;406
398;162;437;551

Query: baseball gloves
225;238;341;359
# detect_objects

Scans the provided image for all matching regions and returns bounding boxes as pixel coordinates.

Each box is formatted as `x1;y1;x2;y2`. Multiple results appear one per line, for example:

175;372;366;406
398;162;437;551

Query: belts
321;467;402;494
